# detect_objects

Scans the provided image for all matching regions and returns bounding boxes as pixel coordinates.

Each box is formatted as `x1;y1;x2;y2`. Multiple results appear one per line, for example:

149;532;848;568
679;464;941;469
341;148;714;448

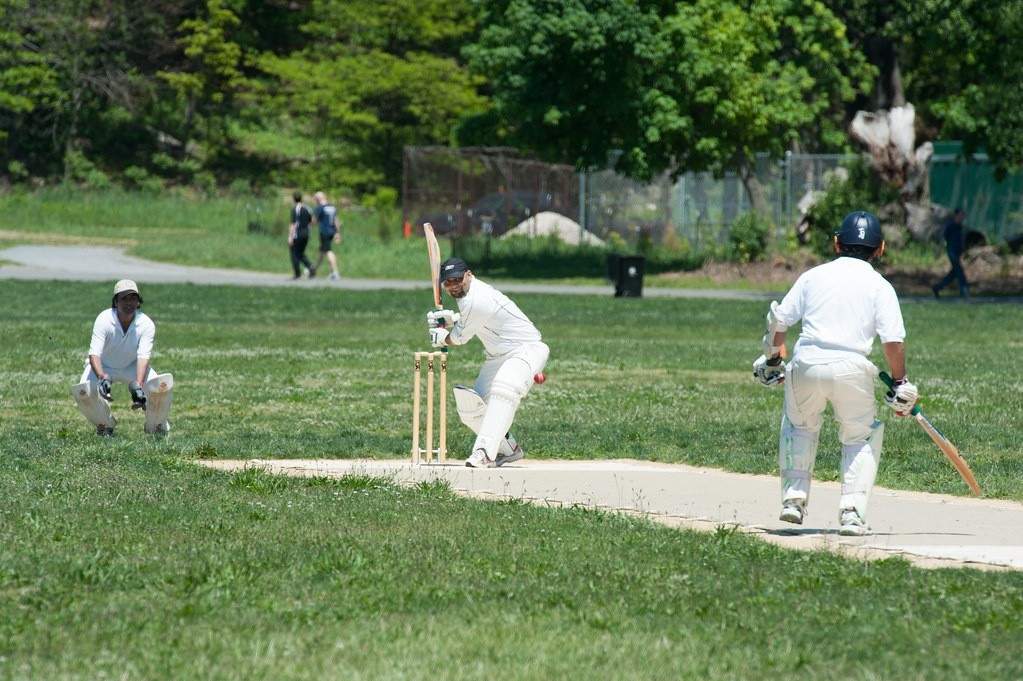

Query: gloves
429;328;449;347
884;376;918;415
99;374;114;402
427;310;454;328
129;380;147;411
753;354;786;387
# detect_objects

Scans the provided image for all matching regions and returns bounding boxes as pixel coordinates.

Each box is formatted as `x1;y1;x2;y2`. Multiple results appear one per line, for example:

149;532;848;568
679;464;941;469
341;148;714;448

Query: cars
412;189;561;241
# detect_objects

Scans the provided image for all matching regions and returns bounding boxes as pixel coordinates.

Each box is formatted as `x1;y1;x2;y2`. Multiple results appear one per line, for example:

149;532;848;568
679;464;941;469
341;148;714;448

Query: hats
440;258;468;283
113;279;138;299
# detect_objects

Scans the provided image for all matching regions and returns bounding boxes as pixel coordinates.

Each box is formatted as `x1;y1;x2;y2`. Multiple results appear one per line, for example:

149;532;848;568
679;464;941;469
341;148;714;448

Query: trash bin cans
616;257;647;298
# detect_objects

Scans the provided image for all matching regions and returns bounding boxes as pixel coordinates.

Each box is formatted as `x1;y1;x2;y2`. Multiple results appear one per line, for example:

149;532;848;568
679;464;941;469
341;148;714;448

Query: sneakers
780;499;807;525
839;510;872;535
495;444;524;466
466;448;496;468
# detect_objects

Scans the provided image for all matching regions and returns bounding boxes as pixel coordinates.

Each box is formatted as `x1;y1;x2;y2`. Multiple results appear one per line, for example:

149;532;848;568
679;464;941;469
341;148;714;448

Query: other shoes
325;272;340;281
289;273;301;281
307;267;316;279
97;428;114;438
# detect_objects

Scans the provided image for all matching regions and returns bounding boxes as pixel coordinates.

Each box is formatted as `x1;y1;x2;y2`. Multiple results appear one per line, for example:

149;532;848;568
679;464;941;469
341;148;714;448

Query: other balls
534;373;546;384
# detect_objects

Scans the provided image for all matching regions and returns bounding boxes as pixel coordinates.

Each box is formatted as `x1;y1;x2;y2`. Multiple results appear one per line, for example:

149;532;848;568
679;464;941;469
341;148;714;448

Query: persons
761;211;918;536
930;207;971;300
70;279;173;442
426;258;550;468
305;191;340;284
288;191;316;282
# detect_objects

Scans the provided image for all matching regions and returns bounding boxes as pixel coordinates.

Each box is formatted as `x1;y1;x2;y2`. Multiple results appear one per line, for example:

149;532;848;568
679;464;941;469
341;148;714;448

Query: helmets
839;211;883;247
